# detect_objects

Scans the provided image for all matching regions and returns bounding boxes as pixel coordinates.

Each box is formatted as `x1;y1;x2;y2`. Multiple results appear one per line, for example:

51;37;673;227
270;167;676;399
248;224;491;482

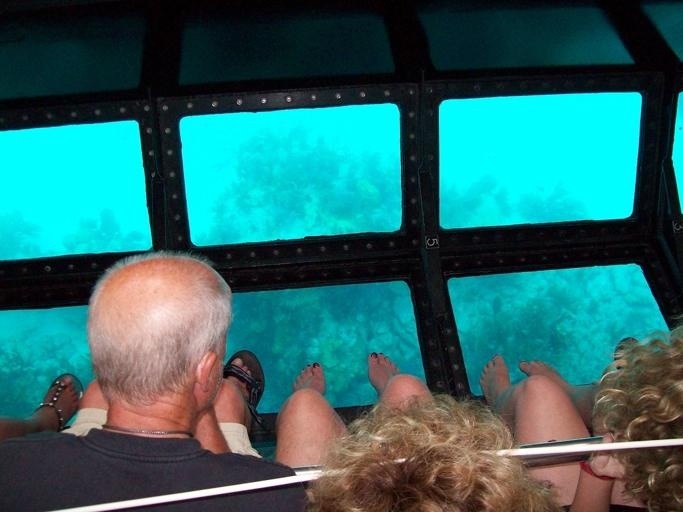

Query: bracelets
577;460;616;484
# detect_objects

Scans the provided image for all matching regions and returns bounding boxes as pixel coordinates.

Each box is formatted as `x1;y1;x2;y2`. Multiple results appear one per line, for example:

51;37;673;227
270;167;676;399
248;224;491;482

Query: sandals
30;373;84;431
222;350;272;444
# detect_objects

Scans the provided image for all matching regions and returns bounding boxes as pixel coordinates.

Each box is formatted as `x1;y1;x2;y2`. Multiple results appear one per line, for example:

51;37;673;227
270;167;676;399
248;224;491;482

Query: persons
476;321;682;512
613;336;645;372
269;350;564;511
0;371;85;439
0;248;316;512
